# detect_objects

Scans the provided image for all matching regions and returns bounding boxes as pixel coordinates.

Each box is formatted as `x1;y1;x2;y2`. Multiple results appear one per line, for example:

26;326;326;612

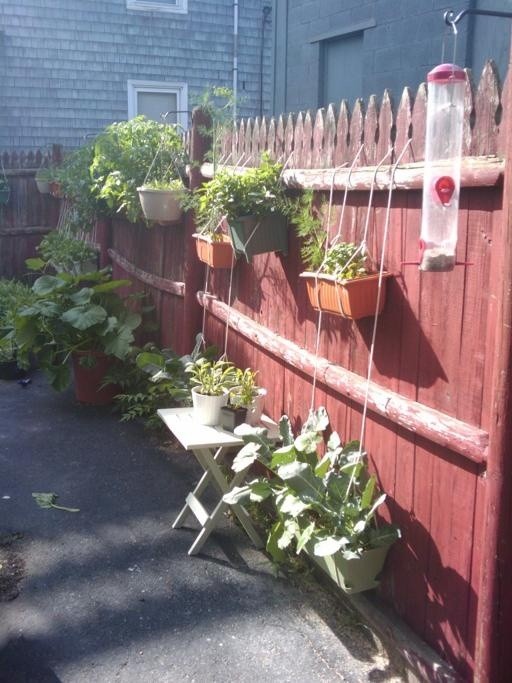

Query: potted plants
176;355;228;427
185;190;240;271
195;148;298;266
284;185;390;324
134;173;187;222
1;119;189;421
227;366;270;429
223;404;399;594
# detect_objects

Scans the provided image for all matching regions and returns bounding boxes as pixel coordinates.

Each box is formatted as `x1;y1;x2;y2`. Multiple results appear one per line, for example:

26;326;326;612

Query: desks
155;405;287;560
